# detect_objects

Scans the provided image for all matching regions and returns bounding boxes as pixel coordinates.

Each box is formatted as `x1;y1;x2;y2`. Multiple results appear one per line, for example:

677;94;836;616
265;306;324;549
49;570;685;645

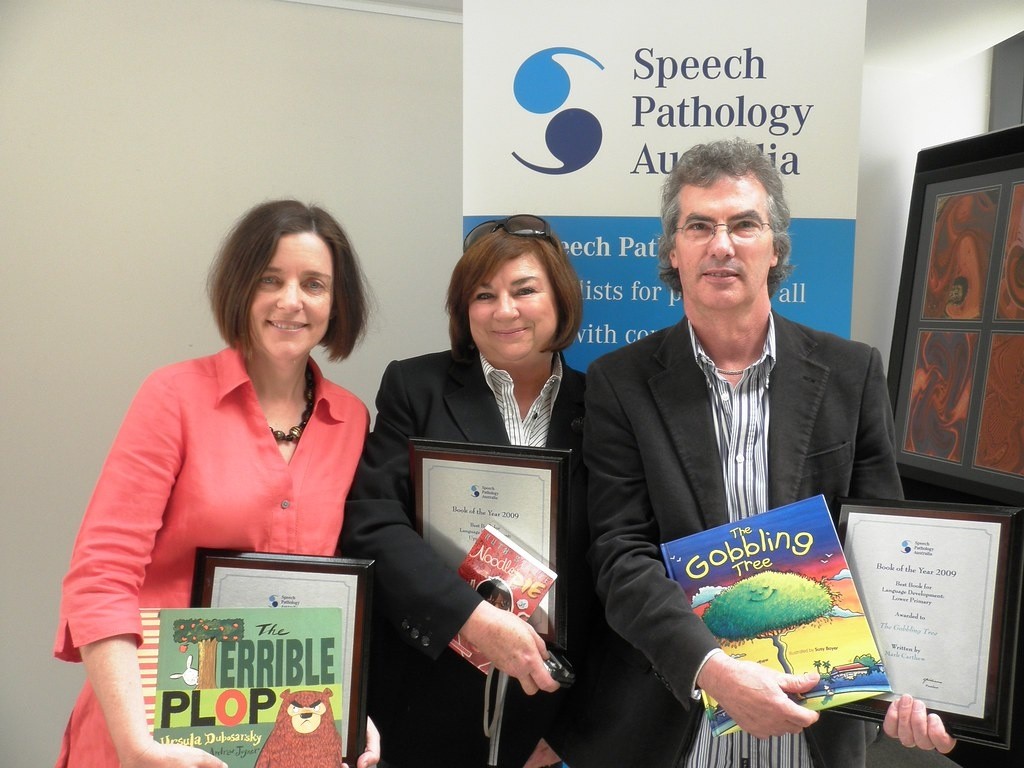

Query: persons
54;198;379;768
339;215;585;768
584;139;957;768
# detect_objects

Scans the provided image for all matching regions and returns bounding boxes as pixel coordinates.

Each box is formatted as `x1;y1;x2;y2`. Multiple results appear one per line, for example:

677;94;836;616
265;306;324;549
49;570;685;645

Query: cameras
540;648;578;688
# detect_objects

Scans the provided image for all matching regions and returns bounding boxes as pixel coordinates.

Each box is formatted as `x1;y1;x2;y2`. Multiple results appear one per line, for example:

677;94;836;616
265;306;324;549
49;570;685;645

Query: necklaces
270;362;316;442
717;369;744;376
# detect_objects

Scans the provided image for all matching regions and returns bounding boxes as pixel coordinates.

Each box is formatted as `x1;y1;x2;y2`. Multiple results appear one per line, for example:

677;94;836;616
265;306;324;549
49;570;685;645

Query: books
448;524;559;675
660;493;891;737
155;607;344;768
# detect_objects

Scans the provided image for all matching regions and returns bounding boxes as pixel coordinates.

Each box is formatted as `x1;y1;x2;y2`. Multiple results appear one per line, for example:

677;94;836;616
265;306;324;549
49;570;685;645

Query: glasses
462;214;558;252
671;216;772;244
